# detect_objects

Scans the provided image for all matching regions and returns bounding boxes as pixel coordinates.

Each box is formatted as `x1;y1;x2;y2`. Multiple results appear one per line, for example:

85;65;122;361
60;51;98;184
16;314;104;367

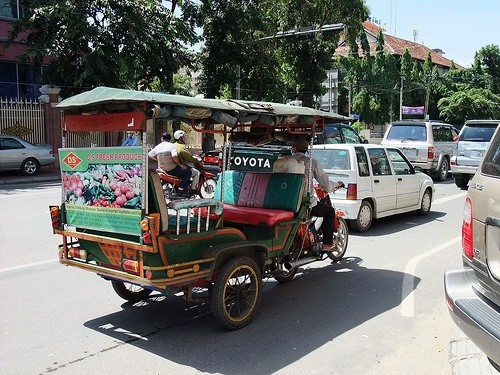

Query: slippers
321;245;339;251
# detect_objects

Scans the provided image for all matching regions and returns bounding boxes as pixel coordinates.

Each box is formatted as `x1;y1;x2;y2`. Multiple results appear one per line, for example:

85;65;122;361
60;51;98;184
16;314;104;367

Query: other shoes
177;189;186;197
191;189;201;196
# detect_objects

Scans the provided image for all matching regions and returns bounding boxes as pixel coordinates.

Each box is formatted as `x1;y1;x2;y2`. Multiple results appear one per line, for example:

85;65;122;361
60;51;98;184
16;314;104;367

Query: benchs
147;168;224;241
192;170;306;232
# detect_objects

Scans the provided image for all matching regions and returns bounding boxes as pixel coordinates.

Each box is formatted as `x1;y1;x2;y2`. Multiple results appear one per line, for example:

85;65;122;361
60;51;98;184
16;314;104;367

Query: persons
121;131;140;147
148;128;204;196
289;139;345;251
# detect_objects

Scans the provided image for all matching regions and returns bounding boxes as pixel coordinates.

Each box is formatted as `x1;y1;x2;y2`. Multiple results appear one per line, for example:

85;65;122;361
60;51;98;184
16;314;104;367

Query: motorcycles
49;84;359;332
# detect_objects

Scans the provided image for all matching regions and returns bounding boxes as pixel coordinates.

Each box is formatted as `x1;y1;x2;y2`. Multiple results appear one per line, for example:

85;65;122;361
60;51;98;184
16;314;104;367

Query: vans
303;141;435;233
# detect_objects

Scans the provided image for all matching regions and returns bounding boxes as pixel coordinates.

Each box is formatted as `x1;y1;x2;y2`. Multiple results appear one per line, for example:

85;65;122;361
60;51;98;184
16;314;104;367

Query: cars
324;123;369;145
0;135;57;177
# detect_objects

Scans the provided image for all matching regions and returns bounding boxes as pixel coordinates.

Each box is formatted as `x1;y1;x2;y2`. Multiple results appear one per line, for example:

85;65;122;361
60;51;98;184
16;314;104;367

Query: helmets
174;130;185;139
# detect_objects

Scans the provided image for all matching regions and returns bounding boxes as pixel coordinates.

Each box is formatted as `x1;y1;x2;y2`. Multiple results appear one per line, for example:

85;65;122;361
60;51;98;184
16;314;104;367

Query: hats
293;141;309;150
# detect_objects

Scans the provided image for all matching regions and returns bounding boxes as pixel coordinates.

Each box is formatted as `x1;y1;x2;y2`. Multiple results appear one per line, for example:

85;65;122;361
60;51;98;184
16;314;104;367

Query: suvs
443;120;500;372
379;118;461;183
447;118;500;188
